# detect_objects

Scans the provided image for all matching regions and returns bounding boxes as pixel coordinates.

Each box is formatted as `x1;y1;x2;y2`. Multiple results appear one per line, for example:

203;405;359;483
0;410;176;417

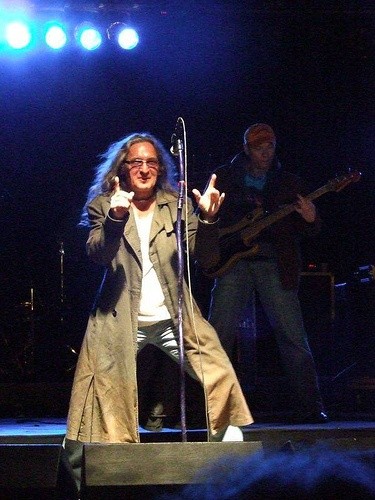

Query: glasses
124;158;158;166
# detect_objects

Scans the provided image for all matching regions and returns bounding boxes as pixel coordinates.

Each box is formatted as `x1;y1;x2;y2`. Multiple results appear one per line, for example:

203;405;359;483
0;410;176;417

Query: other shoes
302;412;327;424
176;411;207;429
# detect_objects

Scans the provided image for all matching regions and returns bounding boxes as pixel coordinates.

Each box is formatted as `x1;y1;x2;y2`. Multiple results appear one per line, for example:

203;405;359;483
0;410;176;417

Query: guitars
191;166;363;279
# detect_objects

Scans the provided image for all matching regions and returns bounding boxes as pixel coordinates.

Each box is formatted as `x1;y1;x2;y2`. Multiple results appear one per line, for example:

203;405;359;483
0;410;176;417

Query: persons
60;136;254;447
193;125;328;425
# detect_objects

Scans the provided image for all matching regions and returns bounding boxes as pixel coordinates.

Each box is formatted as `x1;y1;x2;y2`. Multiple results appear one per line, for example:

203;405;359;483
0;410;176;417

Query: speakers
82;441;264;500
0;443;65;500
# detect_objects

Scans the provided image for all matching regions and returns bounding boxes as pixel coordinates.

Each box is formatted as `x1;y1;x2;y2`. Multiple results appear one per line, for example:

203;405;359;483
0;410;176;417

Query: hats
245;123;276;143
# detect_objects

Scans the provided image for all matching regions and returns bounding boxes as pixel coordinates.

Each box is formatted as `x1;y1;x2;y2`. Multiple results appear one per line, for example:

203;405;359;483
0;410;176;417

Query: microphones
170;117;184;155
